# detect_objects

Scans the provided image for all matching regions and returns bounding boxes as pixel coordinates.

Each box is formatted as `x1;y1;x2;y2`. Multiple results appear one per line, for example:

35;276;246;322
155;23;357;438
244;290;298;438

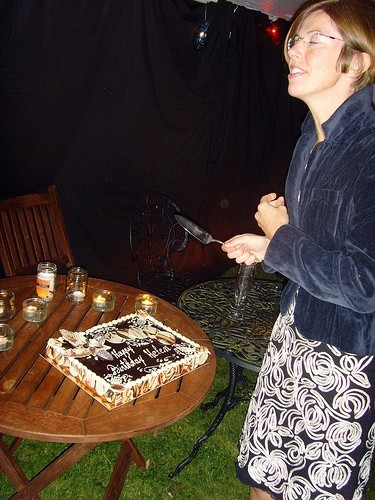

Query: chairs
128;191;201;307
0;184;75;277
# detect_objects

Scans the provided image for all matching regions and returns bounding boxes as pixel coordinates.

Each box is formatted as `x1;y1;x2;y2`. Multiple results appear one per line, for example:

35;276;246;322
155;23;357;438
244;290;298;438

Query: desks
0;275;216;500
169;279;286;479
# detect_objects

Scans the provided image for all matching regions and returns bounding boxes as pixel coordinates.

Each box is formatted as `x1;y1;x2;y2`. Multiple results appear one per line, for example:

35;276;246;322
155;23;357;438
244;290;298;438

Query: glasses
288;30;364;53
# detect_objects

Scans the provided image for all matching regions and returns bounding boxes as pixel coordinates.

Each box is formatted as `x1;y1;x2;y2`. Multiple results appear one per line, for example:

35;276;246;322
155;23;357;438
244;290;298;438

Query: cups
65;266;88;305
0;323;15;351
230;262;258;310
0;289;16;321
35;262;57;301
92;290;116;312
134;293;160;315
22;298;48;323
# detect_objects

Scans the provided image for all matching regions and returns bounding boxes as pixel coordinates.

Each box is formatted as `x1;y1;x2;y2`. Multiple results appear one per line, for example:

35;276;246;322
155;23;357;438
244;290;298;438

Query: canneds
35;262;57;302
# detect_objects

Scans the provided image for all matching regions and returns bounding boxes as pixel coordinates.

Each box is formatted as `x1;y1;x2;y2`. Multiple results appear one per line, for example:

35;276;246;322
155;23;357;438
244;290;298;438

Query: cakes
44;310;209;408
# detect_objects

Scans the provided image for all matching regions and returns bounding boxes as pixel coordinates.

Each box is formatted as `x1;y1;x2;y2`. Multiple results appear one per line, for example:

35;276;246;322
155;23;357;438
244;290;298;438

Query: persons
221;0;375;500
